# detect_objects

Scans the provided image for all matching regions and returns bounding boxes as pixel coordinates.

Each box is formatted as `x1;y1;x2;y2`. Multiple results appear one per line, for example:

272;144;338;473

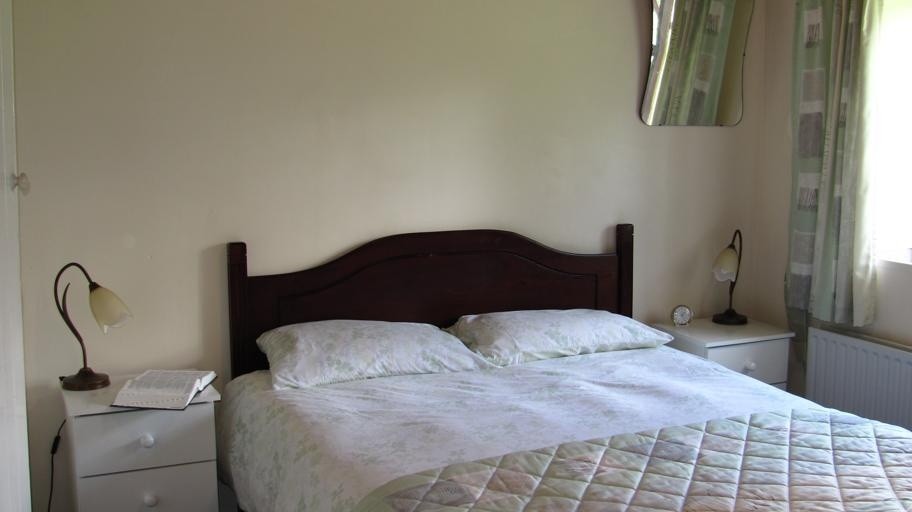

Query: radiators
805;326;912;431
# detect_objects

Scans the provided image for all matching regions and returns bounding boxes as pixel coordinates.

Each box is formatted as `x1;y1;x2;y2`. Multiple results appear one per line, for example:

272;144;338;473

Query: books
108;367;219;413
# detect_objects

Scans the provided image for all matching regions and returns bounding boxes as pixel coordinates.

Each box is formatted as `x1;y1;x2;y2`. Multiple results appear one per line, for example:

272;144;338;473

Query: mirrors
637;1;757;127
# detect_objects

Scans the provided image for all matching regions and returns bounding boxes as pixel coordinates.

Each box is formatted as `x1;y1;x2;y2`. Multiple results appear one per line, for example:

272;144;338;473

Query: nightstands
56;369;223;511
652;316;796;393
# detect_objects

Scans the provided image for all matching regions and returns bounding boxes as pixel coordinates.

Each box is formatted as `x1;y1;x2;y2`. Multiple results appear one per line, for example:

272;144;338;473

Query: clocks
670;304;694;327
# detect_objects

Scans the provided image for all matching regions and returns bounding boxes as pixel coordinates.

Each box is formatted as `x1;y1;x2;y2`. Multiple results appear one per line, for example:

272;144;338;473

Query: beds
225;223;912;512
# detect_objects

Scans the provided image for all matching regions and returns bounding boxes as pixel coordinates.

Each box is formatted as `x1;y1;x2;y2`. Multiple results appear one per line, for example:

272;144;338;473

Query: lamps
52;261;134;392
710;229;750;325
